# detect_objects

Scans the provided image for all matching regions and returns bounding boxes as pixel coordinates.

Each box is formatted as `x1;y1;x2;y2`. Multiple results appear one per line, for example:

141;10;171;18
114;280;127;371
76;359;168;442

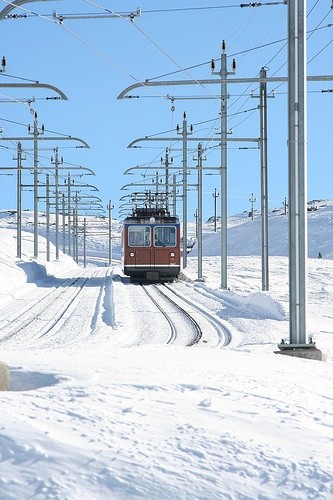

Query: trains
121;208;182;288
